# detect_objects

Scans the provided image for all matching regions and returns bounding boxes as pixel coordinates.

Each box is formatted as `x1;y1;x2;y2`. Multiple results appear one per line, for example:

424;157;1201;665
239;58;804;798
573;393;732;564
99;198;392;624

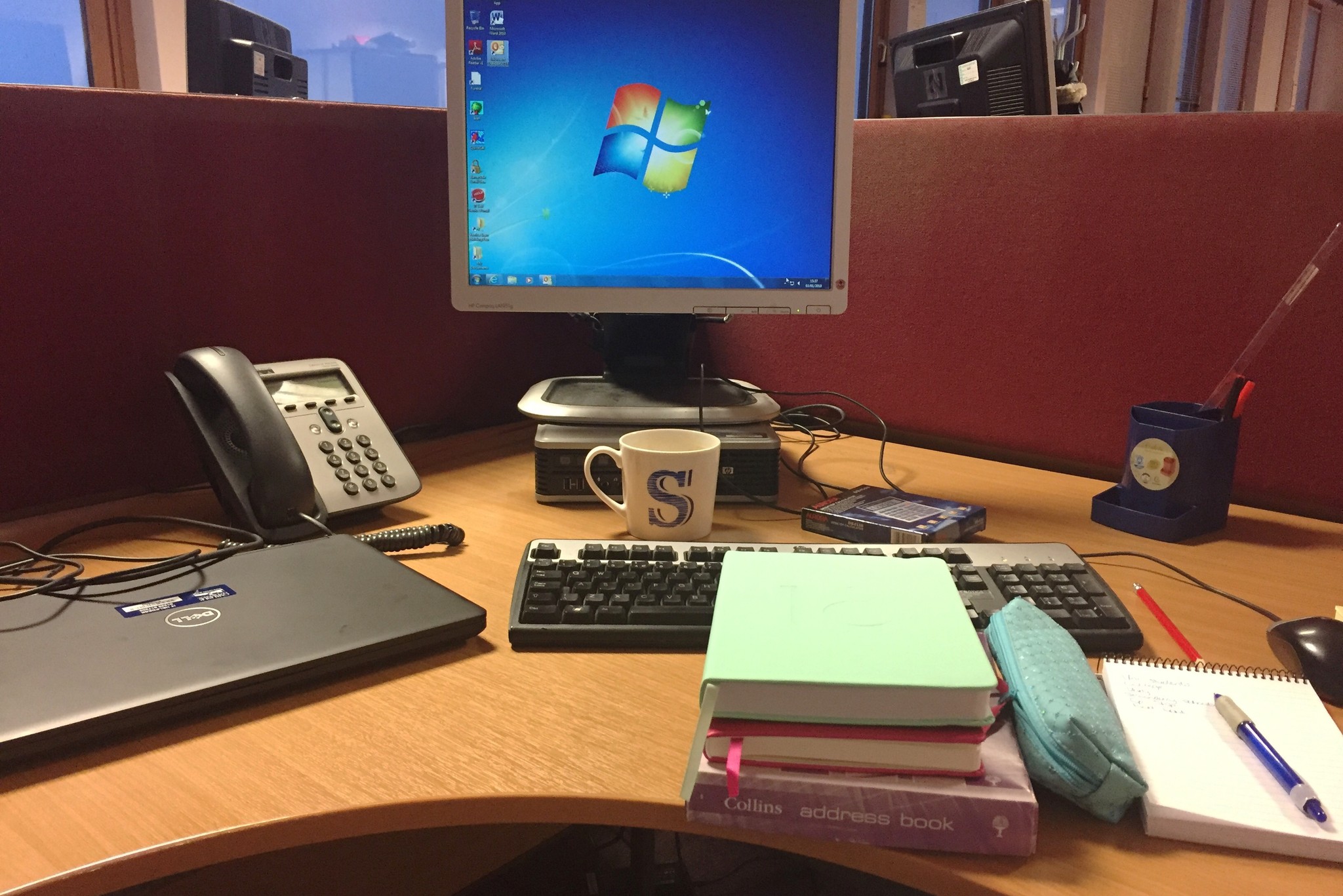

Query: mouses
1265;612;1342;709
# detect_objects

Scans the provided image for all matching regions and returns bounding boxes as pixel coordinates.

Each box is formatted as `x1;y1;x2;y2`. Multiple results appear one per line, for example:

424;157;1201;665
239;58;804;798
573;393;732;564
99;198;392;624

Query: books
698;719;988;780
698;550;1000;728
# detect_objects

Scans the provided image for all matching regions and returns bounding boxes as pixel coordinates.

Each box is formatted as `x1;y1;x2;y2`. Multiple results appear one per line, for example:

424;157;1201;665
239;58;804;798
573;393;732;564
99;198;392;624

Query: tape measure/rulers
1198;222;1343;413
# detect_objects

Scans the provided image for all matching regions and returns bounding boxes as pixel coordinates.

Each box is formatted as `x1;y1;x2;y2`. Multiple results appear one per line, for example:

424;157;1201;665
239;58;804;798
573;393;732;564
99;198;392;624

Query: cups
583;430;720;541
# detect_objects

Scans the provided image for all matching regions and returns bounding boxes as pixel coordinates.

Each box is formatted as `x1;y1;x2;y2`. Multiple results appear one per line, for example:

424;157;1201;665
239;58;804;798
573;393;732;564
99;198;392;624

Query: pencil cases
984;595;1149;827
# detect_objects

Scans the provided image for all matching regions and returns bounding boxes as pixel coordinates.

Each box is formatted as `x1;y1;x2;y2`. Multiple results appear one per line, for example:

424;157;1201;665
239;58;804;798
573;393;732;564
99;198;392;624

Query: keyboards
508;530;1146;662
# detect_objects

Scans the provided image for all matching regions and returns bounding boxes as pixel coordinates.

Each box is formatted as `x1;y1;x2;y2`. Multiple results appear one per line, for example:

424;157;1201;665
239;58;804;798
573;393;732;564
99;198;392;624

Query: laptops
3;526;489;770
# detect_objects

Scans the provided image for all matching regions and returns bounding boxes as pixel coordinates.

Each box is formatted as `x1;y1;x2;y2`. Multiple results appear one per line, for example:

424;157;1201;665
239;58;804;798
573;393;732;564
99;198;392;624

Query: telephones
163;345;423;547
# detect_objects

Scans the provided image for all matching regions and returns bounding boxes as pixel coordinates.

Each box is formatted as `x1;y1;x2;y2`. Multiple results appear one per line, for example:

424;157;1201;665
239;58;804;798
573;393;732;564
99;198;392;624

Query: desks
1;421;1343;896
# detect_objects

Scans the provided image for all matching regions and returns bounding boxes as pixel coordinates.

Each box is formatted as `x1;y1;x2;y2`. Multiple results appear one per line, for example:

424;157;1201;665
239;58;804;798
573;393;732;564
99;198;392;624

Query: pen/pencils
1132;581;1207;671
1219;375;1255;423
1212;692;1327;823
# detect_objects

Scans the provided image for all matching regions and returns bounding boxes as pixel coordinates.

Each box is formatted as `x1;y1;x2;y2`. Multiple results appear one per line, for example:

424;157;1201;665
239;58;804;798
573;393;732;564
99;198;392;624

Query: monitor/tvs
443;2;859;317
888;1;1058;114
183;2;309;99
